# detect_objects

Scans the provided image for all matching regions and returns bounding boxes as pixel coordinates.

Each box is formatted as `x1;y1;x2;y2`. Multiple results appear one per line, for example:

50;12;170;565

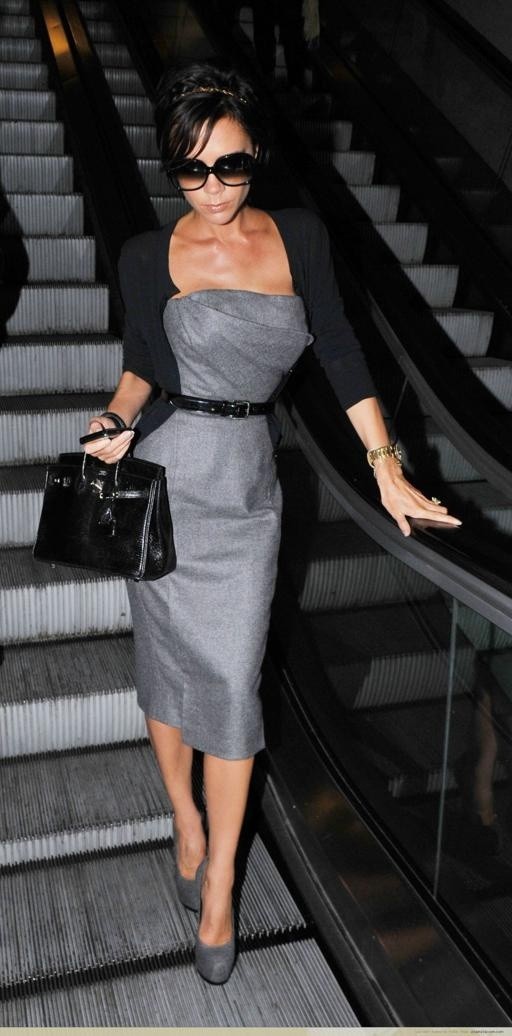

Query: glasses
167;147;263;191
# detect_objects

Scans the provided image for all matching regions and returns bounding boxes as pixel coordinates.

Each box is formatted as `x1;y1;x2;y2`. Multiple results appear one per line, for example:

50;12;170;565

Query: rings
432;497;442;505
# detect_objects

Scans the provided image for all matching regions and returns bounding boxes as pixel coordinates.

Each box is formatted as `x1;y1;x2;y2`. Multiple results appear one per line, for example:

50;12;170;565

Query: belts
160;390;275;419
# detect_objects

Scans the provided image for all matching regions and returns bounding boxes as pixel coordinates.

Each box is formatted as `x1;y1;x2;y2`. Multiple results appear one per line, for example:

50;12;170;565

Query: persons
81;60;462;984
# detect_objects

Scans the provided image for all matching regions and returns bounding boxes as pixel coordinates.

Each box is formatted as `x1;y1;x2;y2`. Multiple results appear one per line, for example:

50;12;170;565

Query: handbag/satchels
34;412;176;581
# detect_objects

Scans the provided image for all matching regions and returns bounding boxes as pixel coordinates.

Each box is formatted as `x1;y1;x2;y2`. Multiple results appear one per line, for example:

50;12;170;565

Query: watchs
367;444;403;469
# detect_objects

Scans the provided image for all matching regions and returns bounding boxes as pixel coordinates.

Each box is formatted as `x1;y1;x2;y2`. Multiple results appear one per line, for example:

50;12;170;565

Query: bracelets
101;412;126;428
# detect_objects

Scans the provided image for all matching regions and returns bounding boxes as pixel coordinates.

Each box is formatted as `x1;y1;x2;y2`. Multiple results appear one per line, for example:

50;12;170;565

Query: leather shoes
172;817;200;912
194;856;235;984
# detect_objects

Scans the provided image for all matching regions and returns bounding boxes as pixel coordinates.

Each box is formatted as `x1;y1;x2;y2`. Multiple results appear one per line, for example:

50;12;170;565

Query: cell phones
79;426;132;445
